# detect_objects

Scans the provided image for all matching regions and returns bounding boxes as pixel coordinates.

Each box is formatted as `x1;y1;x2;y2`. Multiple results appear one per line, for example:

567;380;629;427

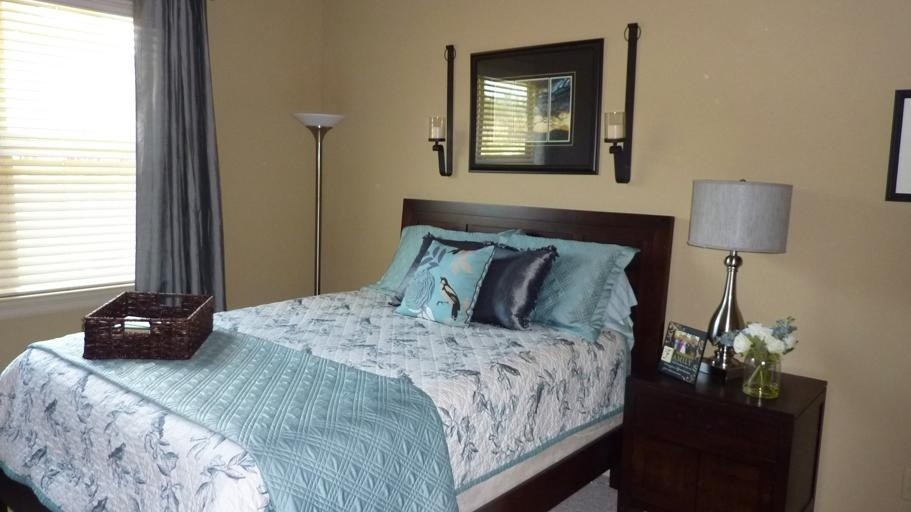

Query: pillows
360;224;641;343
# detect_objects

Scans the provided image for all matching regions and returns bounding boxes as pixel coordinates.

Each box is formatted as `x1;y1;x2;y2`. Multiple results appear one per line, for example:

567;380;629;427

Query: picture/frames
467;37;604;175
885;89;911;202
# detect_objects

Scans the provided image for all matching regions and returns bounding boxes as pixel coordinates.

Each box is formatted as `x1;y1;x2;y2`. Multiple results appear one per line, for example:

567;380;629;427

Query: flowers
720;316;799;387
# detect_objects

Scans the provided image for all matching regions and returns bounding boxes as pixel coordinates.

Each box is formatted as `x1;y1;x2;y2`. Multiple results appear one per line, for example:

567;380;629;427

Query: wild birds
436;276;460;321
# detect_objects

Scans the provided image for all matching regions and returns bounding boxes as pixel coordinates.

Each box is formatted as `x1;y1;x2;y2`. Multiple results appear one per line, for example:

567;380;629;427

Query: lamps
429;44;455;176
294;113;344;295
687;178;793;380
604;22;641;184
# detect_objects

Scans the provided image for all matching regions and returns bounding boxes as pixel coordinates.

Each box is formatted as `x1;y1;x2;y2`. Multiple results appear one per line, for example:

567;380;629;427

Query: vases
742;354;780;399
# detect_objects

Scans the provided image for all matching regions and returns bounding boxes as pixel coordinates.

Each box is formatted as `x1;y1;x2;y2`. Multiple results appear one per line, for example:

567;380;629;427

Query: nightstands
617;358;828;512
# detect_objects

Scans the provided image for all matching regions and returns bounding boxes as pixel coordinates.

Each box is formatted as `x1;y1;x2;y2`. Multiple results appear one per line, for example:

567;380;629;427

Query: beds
0;198;675;512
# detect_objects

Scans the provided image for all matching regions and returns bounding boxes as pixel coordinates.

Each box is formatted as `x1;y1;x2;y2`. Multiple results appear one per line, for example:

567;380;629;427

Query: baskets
80;289;216;361
